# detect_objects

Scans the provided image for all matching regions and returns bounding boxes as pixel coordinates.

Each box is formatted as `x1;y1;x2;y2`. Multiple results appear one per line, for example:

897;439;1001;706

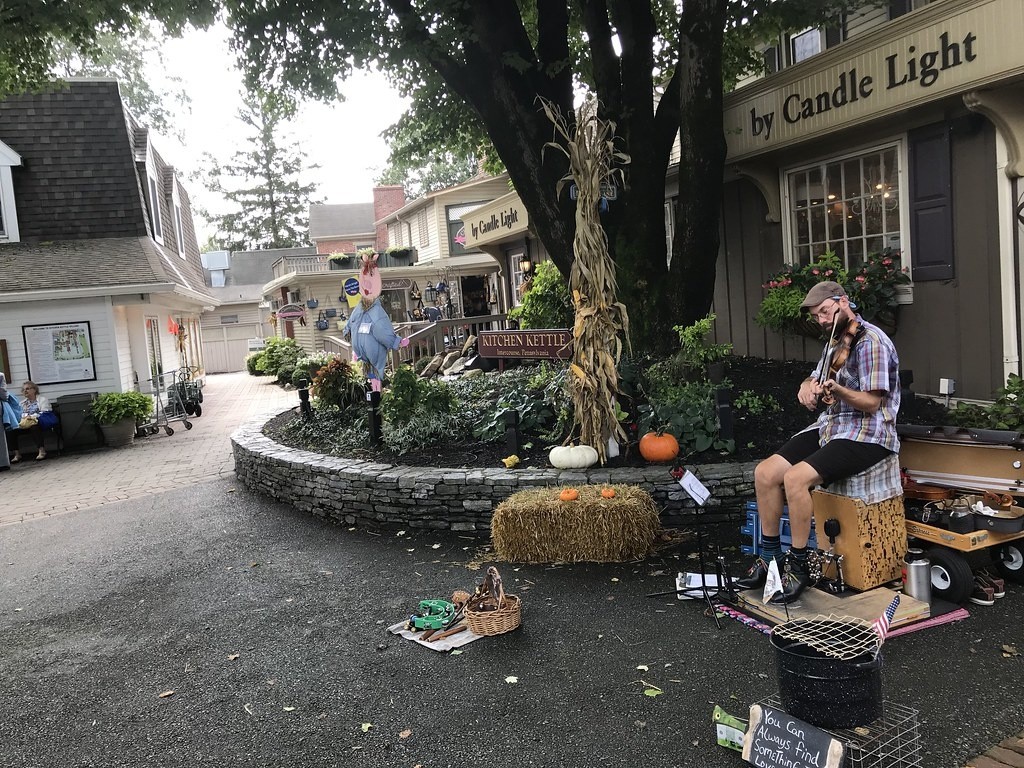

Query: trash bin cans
58;392;103;450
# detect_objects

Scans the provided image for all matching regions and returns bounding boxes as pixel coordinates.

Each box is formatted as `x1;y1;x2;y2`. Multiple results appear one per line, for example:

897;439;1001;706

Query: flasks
901;547;932;608
949;504;975;535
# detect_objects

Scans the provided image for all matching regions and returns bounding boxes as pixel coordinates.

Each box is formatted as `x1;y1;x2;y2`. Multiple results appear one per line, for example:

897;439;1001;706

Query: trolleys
142;365;205;437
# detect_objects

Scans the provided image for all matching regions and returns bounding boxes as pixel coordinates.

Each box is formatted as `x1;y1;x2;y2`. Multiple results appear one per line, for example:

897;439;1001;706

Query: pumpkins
559;489;578;501
602;487;615;497
549;442;598;469
639;428;679;461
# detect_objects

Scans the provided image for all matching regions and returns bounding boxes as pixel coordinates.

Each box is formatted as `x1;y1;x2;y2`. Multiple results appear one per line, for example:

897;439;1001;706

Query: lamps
876;177;887;189
175;324;183;335
519;253;536;281
425;282;438;302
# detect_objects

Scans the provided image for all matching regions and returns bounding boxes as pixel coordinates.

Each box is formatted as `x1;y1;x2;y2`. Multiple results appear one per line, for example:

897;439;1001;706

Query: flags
871;597;903;660
168;316;178;336
763;560;783;604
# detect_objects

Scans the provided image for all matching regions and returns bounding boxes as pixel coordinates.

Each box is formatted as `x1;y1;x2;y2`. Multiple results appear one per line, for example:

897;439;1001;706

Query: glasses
810;299;839;323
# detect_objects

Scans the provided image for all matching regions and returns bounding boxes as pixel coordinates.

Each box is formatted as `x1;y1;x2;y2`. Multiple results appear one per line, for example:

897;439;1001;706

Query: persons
11;382;52;463
0;372;22;470
738;281;901;596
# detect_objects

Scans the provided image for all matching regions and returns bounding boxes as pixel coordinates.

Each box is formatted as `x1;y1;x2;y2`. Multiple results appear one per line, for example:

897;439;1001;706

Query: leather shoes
771;572;810;605
735;557;784;589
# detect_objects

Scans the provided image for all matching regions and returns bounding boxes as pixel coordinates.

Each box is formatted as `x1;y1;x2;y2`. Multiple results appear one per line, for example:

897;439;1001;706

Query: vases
791;310;896;336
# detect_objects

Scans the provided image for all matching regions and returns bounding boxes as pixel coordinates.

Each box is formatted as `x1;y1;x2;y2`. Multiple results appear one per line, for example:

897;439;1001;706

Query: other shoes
35;452;47;460
11;455;22;463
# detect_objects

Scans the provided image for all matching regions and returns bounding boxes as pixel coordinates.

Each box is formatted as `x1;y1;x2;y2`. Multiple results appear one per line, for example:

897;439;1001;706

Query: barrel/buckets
769;620;883;729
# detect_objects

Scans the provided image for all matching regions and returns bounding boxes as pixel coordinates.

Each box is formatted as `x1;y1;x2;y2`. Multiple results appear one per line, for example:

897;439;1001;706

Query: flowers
752;247;911;336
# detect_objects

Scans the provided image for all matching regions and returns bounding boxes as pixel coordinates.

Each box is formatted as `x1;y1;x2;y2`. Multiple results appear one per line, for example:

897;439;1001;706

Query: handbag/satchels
36;411;59;429
18;415;38;430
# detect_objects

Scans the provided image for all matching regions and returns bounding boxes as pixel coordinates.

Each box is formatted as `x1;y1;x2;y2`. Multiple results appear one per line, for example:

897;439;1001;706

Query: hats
800;281;846;313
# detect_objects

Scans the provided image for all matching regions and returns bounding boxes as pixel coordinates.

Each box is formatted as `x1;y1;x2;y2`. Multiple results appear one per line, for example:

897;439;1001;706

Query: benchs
18;403;60;457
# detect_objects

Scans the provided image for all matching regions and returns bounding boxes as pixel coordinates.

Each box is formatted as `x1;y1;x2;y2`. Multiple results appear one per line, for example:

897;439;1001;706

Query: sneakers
969;576;995;606
971;567;1005;599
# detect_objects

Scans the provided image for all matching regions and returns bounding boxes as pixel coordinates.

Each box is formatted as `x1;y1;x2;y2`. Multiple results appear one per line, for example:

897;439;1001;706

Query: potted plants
385;246;410;257
356;247;379;259
328;252;349;263
88;390;154;447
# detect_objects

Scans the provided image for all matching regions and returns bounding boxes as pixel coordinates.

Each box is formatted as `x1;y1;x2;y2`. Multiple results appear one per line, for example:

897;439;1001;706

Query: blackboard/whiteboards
743;702;830;767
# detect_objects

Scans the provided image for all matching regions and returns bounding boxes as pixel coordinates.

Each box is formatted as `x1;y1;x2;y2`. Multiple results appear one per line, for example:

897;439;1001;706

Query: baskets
462;565;521;636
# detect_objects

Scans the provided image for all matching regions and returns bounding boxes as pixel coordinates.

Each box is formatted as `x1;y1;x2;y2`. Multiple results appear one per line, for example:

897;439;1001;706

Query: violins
821;319;863;405
901;481;1019;508
